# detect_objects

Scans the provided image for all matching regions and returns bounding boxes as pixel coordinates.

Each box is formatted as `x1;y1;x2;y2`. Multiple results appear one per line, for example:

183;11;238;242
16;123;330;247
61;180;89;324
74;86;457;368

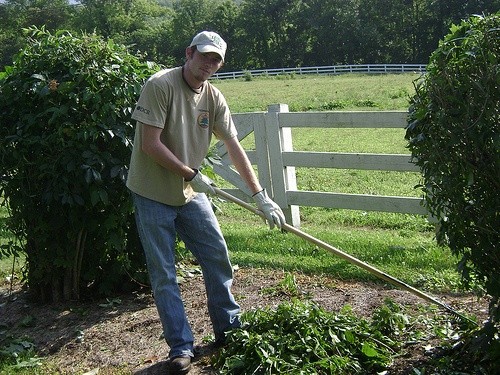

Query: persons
126;31;286;373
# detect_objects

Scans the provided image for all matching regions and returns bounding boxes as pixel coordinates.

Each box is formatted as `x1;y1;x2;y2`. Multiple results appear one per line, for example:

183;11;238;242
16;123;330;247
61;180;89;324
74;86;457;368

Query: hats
190;31;227;63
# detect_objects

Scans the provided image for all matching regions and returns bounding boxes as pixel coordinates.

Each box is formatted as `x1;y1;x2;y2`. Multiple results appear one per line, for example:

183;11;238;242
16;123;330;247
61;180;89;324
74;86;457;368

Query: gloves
251;188;286;230
185;167;217;196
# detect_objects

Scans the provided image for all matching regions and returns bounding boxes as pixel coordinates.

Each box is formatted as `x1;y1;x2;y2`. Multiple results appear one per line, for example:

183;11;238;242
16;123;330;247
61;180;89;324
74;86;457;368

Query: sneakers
171;348;191;373
